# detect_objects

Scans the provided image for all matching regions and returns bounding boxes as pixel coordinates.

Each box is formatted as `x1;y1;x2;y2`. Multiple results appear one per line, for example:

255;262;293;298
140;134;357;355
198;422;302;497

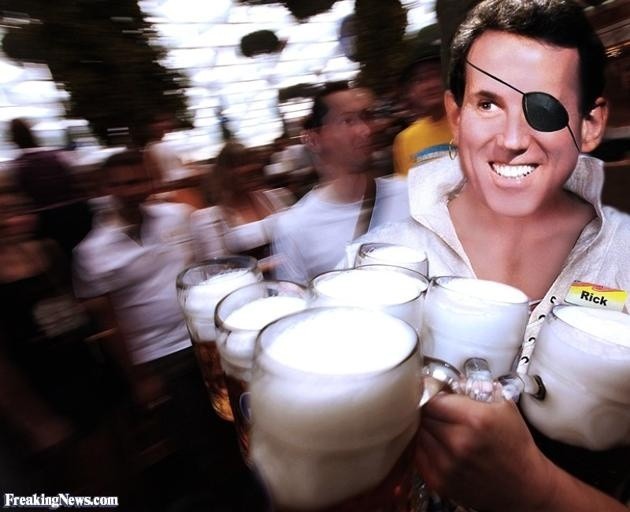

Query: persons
1;0;630;512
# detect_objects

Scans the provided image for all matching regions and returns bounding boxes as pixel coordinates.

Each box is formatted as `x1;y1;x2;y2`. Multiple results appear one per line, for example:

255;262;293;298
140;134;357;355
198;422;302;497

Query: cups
174;236;628;512
